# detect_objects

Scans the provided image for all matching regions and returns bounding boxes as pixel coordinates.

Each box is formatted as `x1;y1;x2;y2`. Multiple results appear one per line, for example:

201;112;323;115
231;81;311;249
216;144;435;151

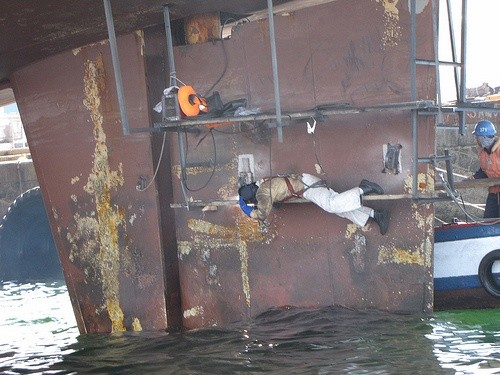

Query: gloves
239;196;254;218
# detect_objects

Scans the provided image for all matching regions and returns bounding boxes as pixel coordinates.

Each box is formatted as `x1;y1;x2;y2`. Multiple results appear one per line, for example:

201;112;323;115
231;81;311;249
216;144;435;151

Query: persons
238;172;389;235
466;120;500;218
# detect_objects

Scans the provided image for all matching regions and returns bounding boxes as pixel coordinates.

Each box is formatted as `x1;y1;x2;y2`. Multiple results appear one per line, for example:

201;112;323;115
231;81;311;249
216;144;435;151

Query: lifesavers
478;249;500;298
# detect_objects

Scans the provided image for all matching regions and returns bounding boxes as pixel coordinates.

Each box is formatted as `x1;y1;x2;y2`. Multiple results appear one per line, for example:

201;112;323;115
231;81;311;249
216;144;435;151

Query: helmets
472;120;497;136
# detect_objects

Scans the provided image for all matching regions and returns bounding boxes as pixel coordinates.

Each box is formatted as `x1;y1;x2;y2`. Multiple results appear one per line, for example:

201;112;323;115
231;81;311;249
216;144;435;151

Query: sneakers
374;209;389;235
359;179;384;195
